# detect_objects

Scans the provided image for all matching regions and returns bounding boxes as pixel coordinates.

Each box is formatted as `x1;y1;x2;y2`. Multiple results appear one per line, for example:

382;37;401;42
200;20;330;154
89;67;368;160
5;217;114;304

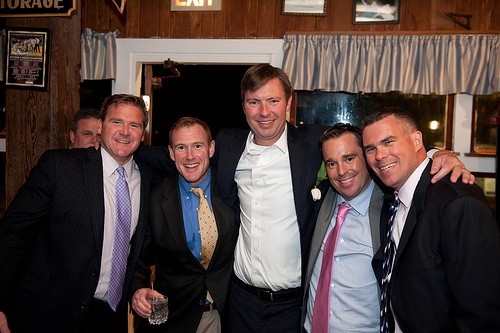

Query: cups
146;295;168;325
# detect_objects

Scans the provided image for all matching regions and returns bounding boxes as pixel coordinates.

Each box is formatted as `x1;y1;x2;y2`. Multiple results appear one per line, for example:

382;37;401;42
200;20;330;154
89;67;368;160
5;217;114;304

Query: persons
96;63;475;333
300;123;386;333
68;108;102;150
0;94;151;333
361;107;500;333
128;117;238;333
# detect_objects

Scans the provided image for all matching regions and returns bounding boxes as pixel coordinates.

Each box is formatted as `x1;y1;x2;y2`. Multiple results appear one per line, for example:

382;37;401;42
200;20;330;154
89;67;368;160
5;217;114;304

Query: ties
380;195;401;333
107;166;132;312
190;187;219;304
311;203;353;333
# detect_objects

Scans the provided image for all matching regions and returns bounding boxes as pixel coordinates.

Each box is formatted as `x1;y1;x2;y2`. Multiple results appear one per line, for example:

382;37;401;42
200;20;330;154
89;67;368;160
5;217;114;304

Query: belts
232;273;303;304
204;302;216;311
